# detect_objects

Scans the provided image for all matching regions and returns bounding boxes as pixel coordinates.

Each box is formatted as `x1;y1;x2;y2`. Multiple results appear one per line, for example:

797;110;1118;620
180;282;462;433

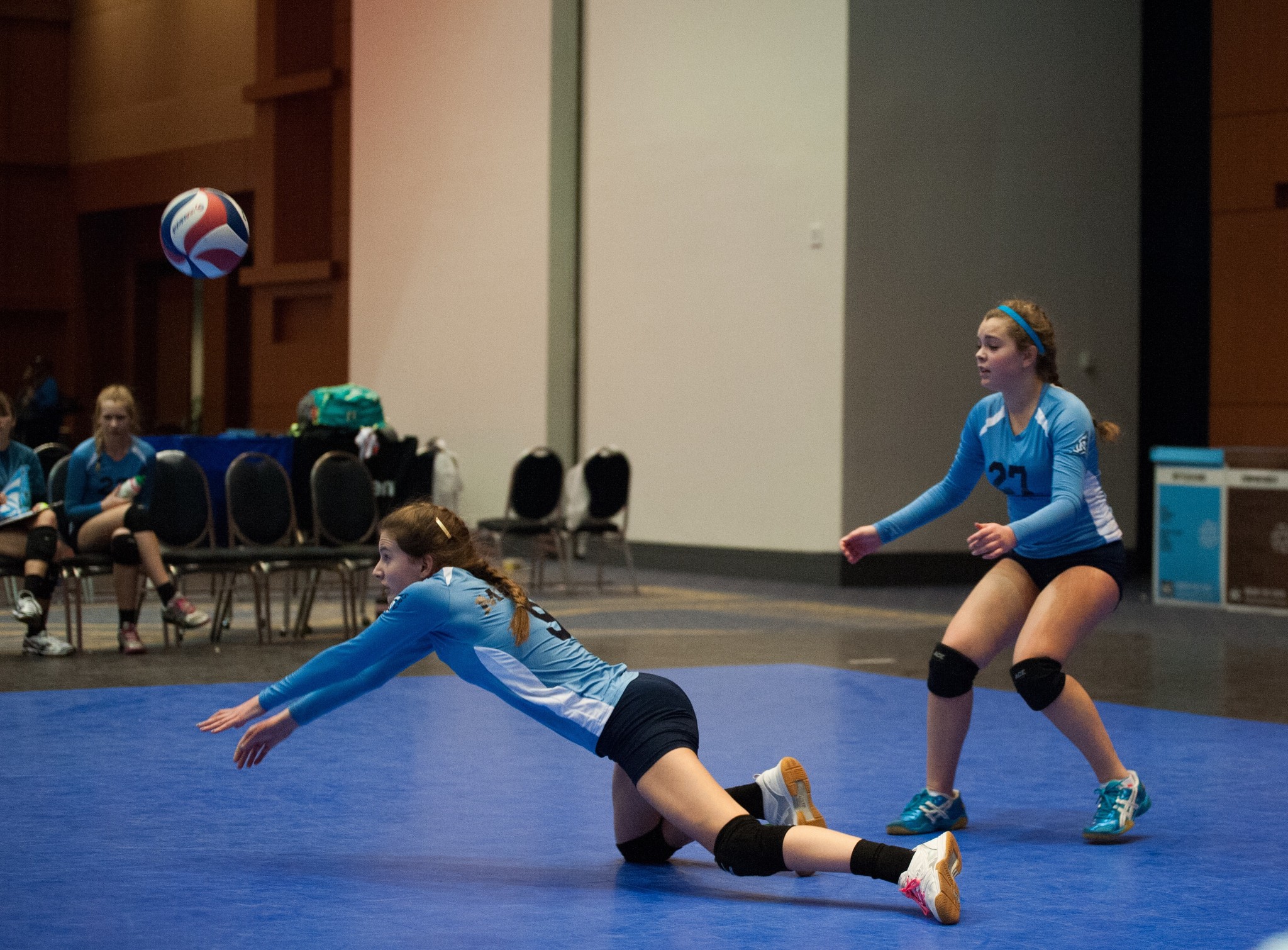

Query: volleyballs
158;187;250;281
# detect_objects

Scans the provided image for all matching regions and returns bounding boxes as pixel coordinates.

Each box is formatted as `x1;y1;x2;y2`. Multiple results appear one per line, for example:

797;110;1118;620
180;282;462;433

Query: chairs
0;442;379;646
478;446;639;597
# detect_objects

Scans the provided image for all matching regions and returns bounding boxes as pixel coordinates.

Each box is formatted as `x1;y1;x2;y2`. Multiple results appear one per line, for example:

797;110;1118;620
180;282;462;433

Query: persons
17;356;65;484
0;394;77;659
840;296;1154;845
61;385;210;655
194;501;963;928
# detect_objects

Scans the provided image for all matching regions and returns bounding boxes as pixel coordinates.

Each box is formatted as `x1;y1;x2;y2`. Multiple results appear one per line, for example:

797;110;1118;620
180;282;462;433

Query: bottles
117;475;147;498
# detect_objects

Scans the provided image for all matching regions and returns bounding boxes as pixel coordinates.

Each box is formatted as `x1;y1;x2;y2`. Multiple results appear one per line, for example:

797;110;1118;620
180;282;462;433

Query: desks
144;435;438;548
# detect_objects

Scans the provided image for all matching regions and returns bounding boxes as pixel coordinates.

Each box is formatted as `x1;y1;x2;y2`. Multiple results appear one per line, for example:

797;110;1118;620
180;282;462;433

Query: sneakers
22;628;75;656
753;756;828;877
160;592;210;629
11;589;43;622
117;621;144;653
1082;770;1152;841
888;787;968;835
897;831;963;923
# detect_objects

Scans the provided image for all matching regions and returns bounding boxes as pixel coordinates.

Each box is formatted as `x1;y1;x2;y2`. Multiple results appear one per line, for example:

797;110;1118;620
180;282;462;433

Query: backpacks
298;384;386;430
